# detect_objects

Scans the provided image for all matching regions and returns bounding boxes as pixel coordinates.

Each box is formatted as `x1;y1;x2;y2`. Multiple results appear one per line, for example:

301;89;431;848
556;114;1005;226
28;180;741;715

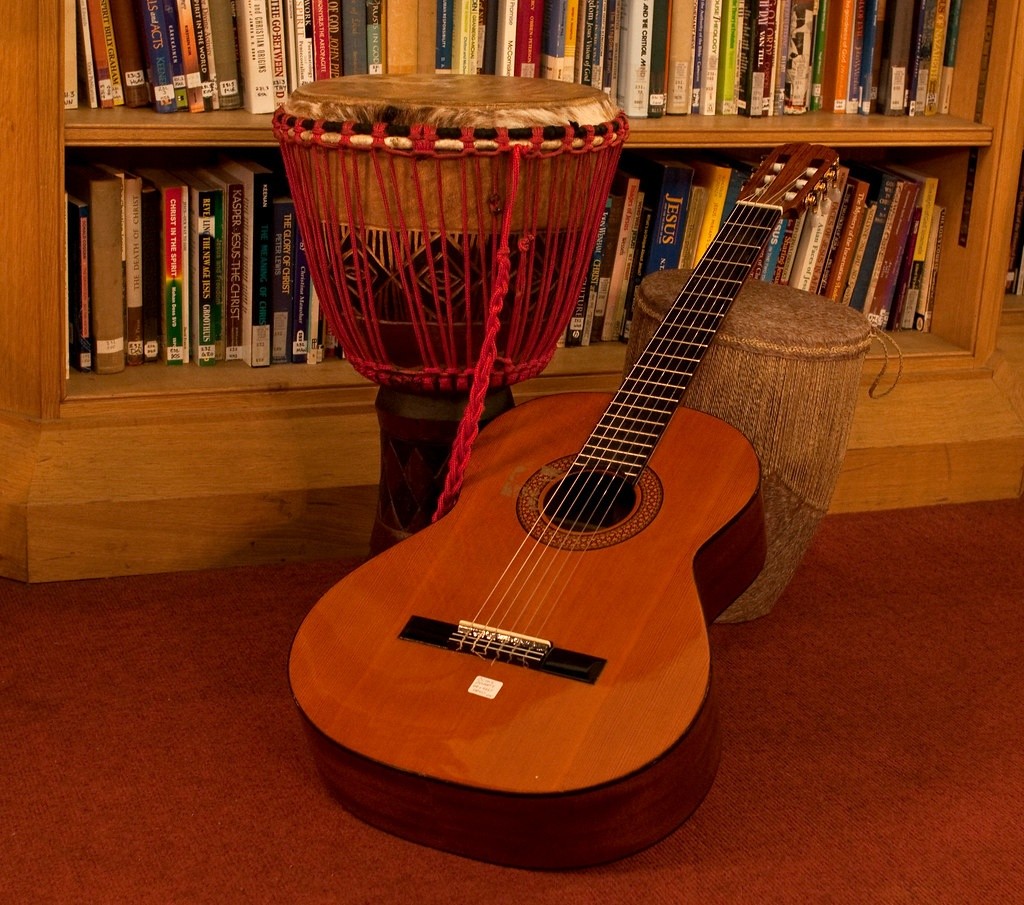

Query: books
63;0;1024;379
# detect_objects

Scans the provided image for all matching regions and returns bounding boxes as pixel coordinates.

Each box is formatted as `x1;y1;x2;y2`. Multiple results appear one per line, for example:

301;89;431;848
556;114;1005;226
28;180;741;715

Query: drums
271;72;632;566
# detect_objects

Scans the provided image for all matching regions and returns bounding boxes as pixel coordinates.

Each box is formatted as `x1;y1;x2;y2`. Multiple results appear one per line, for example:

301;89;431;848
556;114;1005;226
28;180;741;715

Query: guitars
285;139;841;875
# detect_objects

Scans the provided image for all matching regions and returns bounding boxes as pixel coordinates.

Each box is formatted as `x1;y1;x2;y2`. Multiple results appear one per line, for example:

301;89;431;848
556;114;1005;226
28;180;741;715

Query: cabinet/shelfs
0;0;1024;582
